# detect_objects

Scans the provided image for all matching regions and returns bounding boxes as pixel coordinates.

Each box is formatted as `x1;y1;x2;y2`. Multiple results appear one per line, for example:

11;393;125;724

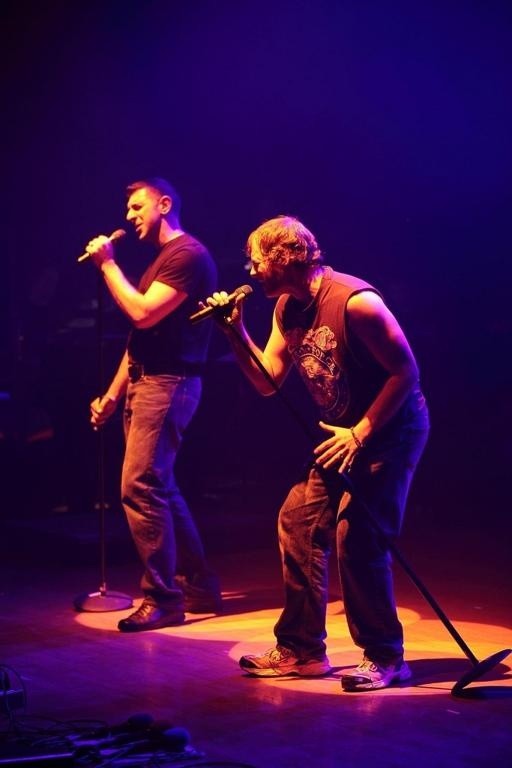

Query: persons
197;215;432;691
85;174;218;630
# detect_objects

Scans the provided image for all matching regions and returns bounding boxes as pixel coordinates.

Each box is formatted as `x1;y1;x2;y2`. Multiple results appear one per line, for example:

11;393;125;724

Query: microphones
189;284;254;324
77;228;128;264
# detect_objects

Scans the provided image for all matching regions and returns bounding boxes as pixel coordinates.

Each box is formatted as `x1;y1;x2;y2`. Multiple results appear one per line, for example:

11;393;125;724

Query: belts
127;361;206;381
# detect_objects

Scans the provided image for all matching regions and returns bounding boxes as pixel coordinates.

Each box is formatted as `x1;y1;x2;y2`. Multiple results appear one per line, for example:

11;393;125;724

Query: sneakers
184;589;223;613
239;642;335;678
341;653;413;691
117;597;185;631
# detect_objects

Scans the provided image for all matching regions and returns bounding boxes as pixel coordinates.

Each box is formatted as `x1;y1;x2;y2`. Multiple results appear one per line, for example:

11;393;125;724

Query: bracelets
349;425;365;452
103;393;118;405
